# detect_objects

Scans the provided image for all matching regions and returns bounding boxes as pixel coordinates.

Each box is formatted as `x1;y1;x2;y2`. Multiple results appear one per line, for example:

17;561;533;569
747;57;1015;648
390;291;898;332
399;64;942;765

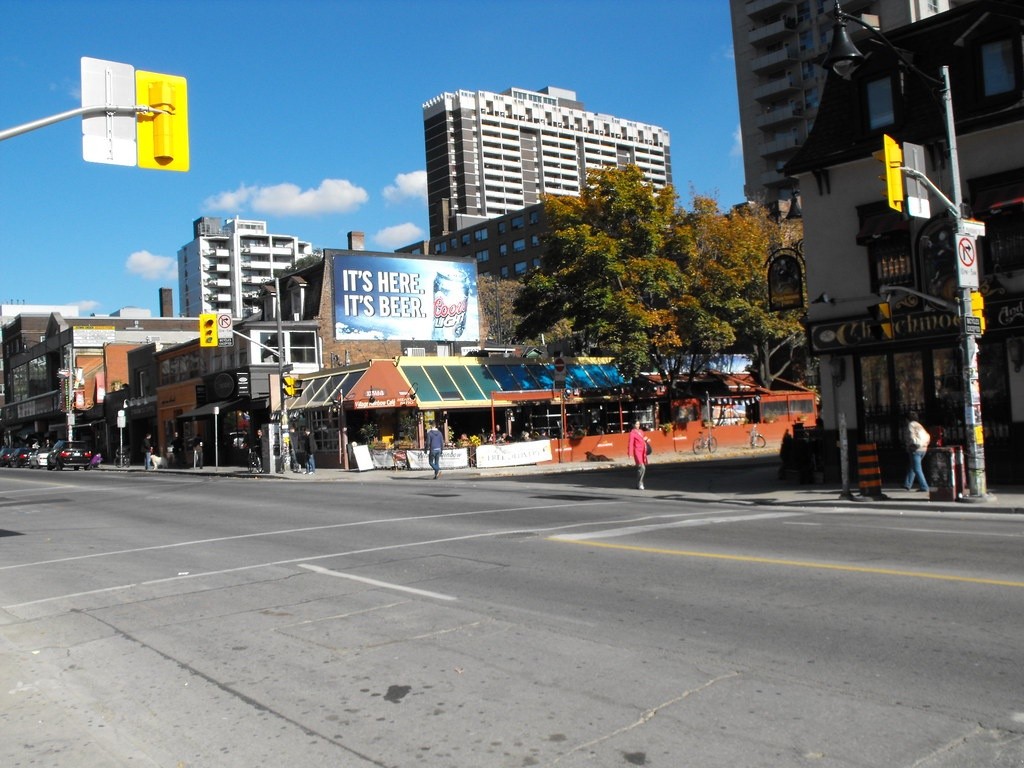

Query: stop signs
554;358;565;371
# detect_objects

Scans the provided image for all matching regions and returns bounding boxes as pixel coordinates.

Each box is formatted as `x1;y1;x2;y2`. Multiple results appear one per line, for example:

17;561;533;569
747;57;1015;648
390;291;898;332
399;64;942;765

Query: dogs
150;455;169;469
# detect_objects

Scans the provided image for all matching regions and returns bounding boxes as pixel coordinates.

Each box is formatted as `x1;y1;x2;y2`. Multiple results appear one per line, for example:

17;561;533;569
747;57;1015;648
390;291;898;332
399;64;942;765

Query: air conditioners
404;348;426;358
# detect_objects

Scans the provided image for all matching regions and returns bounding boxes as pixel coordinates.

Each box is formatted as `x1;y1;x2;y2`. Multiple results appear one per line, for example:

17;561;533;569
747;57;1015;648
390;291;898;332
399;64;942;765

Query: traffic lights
872;133;906;213
198;313;219;348
282;377;295;398
293;378;303;398
866;301;895;342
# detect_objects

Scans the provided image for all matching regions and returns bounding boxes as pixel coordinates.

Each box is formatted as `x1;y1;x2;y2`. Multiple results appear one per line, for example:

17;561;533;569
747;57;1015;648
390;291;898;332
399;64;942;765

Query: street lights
826;1;989;499
20;339;73;445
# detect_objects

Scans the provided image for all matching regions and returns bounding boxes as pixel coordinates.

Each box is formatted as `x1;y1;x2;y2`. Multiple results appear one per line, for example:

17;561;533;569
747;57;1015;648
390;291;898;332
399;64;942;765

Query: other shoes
433;470;442;480
303;471;308;474
309;472;315;475
901;486;909;490
916;488;926;492
639;484;644;490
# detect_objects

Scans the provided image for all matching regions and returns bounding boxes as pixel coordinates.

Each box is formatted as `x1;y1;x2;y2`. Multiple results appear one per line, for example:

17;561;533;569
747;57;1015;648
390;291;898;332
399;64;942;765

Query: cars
28;446;52;468
7;448;35;468
0;447;18;468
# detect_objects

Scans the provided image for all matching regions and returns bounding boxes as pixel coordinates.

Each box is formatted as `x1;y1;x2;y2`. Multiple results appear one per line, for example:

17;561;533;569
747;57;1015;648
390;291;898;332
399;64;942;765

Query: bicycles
247;446;264;473
693;425;718;455
113;449;131;467
747;422;767;451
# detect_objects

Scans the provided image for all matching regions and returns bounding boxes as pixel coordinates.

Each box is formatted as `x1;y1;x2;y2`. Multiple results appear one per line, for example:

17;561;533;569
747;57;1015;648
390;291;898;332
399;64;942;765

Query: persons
91;453;102;468
141;433;153;469
628;420;650;490
255;429;264;473
496;425;510;442
289;427;302;472
424;422;443;479
900;410;930;492
195;441;203;469
303;429;317;475
241;429;253;453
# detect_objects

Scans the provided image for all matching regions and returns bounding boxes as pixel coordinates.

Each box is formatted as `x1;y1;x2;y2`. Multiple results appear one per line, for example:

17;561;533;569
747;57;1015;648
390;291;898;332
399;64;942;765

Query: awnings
177;397;245;467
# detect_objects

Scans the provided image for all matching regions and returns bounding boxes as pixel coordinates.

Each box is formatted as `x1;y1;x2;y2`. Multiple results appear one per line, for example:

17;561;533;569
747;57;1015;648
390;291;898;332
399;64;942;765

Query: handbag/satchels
646;441;652;455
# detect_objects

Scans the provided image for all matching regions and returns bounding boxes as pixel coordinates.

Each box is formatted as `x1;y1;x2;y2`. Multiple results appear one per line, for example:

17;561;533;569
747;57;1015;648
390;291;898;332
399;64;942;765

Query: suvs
46;440;91;470
